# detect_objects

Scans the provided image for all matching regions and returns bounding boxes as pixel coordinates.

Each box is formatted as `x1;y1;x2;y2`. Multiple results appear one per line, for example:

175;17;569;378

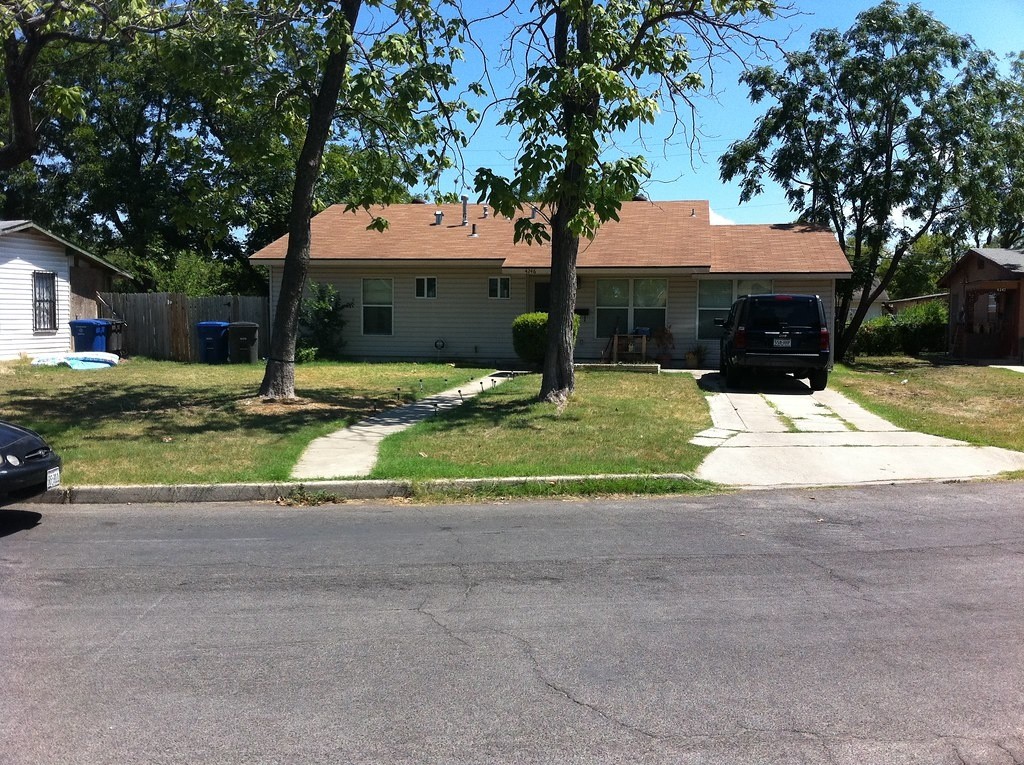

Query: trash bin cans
196;321;229;364
70;317;125;357
229;321;259;364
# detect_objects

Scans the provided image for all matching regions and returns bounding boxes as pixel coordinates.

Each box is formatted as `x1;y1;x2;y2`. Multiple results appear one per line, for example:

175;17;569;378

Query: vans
714;293;830;391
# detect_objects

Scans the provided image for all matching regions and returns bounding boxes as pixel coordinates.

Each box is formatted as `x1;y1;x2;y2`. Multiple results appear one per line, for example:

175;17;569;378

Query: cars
0;419;62;508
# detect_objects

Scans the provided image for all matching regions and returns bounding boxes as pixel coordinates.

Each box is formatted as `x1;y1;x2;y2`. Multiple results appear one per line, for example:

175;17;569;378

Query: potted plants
685;344;711;368
